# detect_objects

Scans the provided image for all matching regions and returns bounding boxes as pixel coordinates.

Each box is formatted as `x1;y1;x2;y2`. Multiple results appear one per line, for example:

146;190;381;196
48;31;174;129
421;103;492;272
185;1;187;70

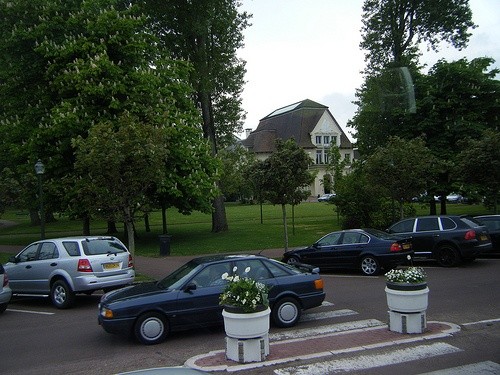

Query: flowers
385;266;425;284
219;267;274;311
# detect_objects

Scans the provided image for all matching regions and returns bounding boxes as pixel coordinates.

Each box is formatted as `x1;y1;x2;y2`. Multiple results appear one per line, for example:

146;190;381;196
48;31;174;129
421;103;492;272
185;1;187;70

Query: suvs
2;235;136;310
382;214;494;267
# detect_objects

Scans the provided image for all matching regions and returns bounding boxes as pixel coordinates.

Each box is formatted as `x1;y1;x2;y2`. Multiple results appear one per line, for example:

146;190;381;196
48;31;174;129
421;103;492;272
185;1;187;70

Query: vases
385;287;429;312
221;307;272;339
223;305;268;313
385;282;427;292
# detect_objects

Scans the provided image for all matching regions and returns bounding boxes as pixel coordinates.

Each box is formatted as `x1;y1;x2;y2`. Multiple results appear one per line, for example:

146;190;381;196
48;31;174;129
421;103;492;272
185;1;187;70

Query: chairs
344;236;352;244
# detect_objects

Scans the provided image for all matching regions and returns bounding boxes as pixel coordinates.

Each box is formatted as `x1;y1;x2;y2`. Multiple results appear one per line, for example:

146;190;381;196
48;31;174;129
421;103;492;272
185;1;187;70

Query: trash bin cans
156;236;172;256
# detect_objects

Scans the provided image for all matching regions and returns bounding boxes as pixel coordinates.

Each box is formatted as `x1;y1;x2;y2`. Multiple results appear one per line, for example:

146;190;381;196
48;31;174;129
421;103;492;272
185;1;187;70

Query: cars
0;263;13;314
472;214;500;253
281;228;416;277
318;193;336;202
97;252;326;345
410;189;475;205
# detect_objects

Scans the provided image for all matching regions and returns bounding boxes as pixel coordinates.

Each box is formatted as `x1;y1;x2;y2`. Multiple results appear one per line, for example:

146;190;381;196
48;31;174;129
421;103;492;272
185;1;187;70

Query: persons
192;265;225;288
